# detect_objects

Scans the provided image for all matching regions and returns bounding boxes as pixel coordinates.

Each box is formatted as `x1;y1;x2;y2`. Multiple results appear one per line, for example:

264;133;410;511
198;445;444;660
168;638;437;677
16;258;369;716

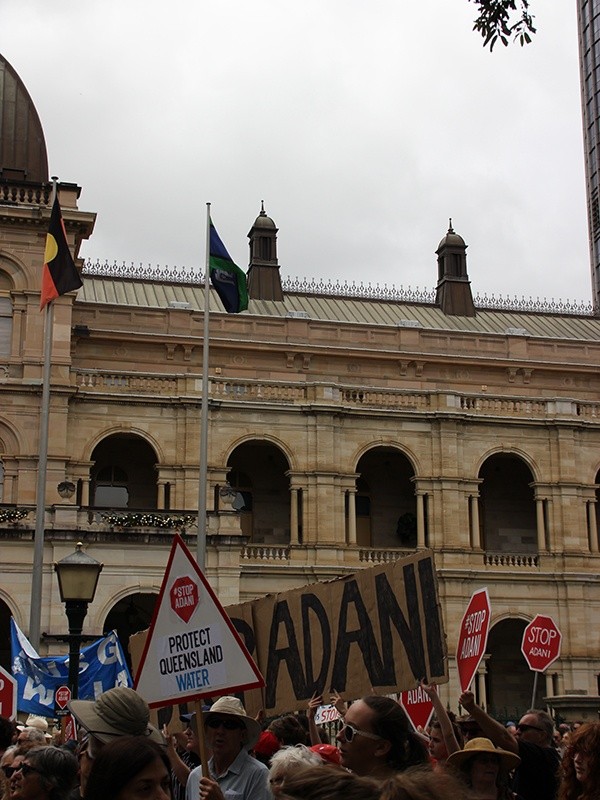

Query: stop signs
521;614;563;671
455;587;491;692
170;577;200;624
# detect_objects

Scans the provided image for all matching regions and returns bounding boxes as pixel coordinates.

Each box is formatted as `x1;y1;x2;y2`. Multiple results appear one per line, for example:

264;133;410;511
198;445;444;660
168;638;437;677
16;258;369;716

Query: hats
503;721;516;728
16;716;53;739
179;712;196;723
189;696;262;751
67;685;165;746
307;743;342;765
445;737;522;787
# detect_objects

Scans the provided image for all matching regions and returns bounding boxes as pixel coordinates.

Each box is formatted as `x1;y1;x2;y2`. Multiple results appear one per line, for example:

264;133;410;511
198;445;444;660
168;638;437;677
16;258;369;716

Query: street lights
54;541;106;702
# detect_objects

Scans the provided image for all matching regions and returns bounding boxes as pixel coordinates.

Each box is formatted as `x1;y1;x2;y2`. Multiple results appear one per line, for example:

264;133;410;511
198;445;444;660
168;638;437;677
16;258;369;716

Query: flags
39;193;84;311
209;216;249;313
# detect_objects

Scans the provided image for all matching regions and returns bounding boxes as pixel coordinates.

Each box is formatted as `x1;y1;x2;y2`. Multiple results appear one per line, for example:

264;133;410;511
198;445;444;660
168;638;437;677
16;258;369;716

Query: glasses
186;722;193;730
206;714;247;730
79;750;95;761
472;756;503;765
18;737;32;745
5;767;20;778
515;723;544;736
337;718;383;744
19;762;43;777
86;728;154;738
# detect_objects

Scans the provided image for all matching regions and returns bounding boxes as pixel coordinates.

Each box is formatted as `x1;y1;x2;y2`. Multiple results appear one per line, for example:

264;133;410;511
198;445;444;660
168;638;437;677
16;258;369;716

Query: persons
0;676;600;800
